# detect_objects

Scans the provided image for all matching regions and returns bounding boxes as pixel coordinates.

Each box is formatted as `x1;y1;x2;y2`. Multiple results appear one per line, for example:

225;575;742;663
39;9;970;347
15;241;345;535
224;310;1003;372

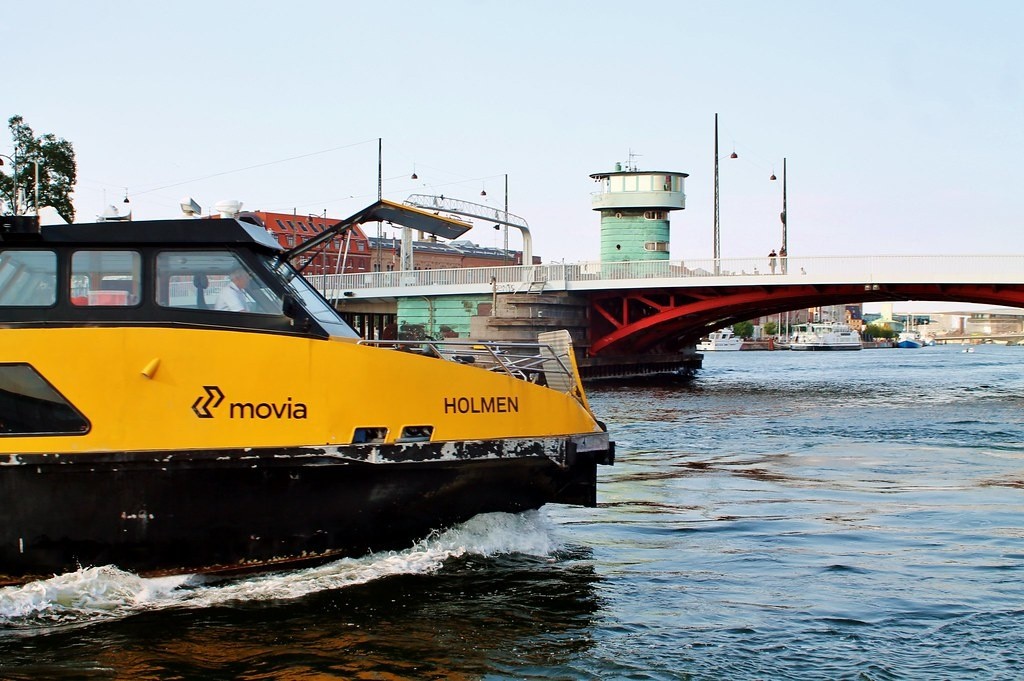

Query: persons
214;267;249;312
767;249;777;274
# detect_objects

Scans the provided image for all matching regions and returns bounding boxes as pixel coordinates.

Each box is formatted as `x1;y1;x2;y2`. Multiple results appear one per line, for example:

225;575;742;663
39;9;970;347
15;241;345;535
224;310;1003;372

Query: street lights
770;164;787;274
27;159;38;215
0;154;16;215
713;149;738;276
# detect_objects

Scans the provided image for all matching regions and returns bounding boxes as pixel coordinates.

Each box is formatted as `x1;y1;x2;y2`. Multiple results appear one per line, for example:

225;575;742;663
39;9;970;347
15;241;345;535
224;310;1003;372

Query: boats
696;326;744;351
898;329;923;347
778;322;864;351
0;198;615;588
961;348;975;353
925;340;936;346
